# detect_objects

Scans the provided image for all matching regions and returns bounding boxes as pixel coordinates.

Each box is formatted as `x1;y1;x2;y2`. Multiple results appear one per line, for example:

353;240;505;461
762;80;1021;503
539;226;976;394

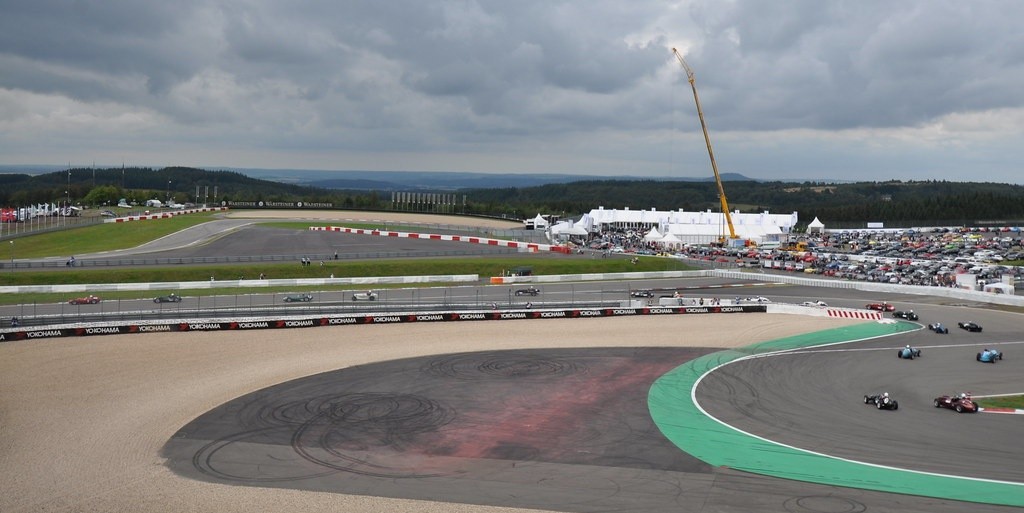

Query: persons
577;228;1024;294
489;285;764;307
9;250;372;328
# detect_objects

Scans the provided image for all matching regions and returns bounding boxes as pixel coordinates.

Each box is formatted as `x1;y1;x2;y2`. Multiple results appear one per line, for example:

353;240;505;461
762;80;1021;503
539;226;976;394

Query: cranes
671;47;753;255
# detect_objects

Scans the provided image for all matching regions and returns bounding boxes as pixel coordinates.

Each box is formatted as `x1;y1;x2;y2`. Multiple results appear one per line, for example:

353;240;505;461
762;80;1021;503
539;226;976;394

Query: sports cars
897;347;921;360
958;321;983;333
892;310;918;321
929;322;949;334
803;299;828;306
282;293;313;302
866;303;896;312
68;295;100;304
352;290;378;301
153;293;183;303
933;395;979;414
515;287;541;297
976;348;1003;363
863;393;899;410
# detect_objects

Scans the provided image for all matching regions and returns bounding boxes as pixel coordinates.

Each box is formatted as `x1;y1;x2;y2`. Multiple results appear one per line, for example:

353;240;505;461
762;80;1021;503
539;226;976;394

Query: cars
564;225;1023;301
1;198;216;222
